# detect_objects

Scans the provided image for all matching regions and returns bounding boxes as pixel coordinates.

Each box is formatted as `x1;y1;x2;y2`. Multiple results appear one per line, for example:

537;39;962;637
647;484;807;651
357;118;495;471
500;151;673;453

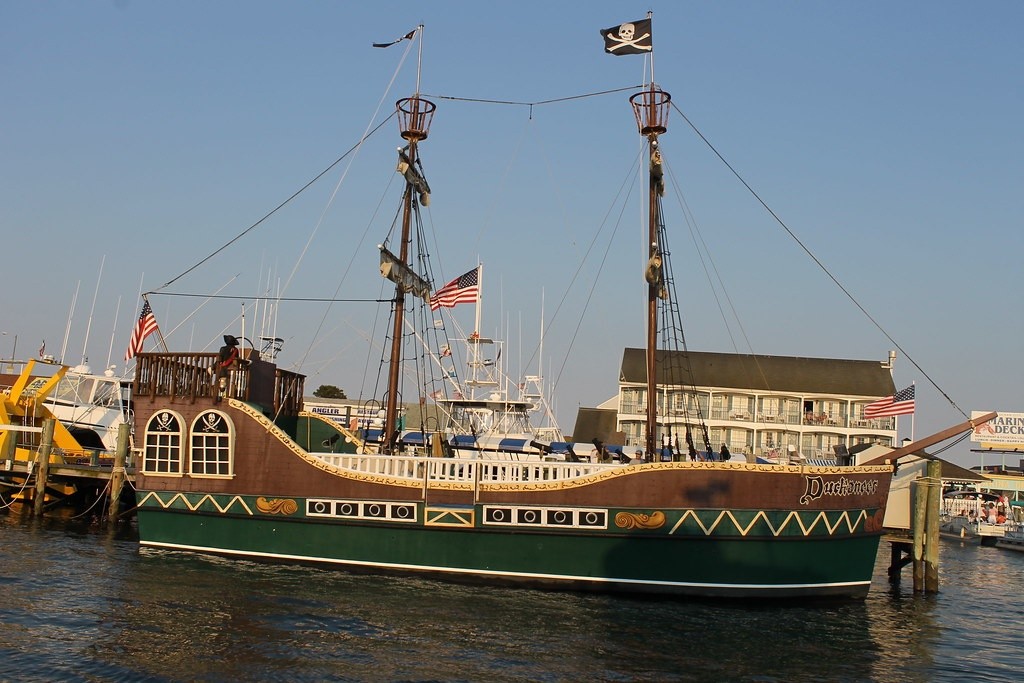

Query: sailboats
125;15;998;601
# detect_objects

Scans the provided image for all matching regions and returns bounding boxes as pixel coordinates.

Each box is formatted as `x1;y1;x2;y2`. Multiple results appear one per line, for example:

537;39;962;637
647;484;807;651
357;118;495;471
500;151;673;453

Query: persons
806;410;828;426
960;501;998;527
629;450;645;466
208;333;241;400
719;442;731;462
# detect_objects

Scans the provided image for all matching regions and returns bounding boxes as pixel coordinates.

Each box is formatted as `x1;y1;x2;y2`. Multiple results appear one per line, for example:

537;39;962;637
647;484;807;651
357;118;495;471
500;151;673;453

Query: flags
39;340;46;357
123;299;159;362
863;384;915;420
600;16;654;57
372;29;416;49
427;266;480;312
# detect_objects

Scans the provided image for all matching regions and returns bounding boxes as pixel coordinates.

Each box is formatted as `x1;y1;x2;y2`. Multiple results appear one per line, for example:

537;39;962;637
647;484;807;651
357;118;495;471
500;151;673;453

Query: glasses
635;453;641;455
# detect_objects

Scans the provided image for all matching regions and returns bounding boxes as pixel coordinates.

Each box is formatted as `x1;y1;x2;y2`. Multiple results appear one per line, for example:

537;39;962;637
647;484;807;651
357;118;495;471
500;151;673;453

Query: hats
223;335;239;346
988;503;994;506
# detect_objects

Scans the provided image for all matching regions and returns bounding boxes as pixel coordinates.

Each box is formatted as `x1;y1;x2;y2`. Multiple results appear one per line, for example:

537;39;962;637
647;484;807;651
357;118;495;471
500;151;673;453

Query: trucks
302;401;408;442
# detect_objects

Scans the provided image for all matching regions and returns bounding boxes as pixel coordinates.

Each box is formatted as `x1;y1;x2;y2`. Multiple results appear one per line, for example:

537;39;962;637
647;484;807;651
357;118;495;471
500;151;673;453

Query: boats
437;253;568;445
0;254;135;468
